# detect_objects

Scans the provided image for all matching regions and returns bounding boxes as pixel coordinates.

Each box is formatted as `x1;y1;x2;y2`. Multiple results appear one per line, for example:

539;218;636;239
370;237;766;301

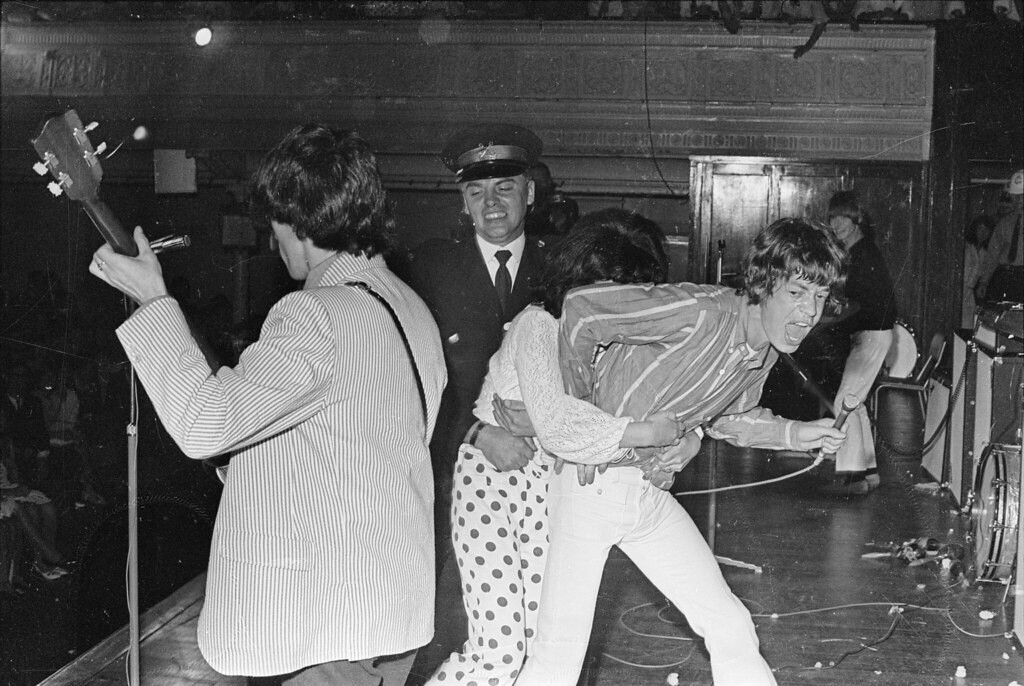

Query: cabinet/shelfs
947;328;1024;514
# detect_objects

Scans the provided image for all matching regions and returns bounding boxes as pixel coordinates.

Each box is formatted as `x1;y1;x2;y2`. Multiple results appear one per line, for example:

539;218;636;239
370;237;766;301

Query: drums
968;442;1022;585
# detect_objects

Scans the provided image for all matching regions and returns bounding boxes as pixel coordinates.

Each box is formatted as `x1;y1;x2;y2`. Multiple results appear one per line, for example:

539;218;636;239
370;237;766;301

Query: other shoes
865;473;880;489
830;479;868;495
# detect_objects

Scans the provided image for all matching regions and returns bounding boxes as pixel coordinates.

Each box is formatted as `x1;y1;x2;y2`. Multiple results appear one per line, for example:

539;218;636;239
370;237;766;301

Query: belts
1002;265;1024;271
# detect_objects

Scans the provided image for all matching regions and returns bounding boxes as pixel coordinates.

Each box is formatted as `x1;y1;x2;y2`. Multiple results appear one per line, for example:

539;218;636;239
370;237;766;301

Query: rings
97;261;106;271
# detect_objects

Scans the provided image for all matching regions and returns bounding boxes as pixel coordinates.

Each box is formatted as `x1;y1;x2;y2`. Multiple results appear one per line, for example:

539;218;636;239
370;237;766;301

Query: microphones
814;394;860;466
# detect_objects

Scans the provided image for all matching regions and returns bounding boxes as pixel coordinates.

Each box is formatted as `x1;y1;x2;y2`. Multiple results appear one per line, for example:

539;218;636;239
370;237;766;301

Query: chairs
870;333;946;445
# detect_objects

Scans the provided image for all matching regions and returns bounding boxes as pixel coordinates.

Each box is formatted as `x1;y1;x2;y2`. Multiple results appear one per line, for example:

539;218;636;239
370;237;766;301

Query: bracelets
469;422;489;446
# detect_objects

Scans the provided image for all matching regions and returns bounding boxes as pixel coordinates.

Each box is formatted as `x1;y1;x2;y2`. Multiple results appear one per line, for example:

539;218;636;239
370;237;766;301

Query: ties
1008;214;1022;264
494;250;512;314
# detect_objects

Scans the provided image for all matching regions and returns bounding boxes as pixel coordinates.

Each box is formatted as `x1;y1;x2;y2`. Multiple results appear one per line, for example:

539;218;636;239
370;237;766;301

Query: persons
89;122;449;686
412;122;1024;686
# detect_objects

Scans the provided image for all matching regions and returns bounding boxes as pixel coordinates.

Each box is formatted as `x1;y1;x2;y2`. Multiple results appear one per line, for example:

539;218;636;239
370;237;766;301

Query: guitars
27;105;229;485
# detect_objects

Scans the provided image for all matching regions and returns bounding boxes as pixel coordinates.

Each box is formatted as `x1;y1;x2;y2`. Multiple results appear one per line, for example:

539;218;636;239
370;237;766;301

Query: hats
1005;170;1024;194
440;122;543;184
